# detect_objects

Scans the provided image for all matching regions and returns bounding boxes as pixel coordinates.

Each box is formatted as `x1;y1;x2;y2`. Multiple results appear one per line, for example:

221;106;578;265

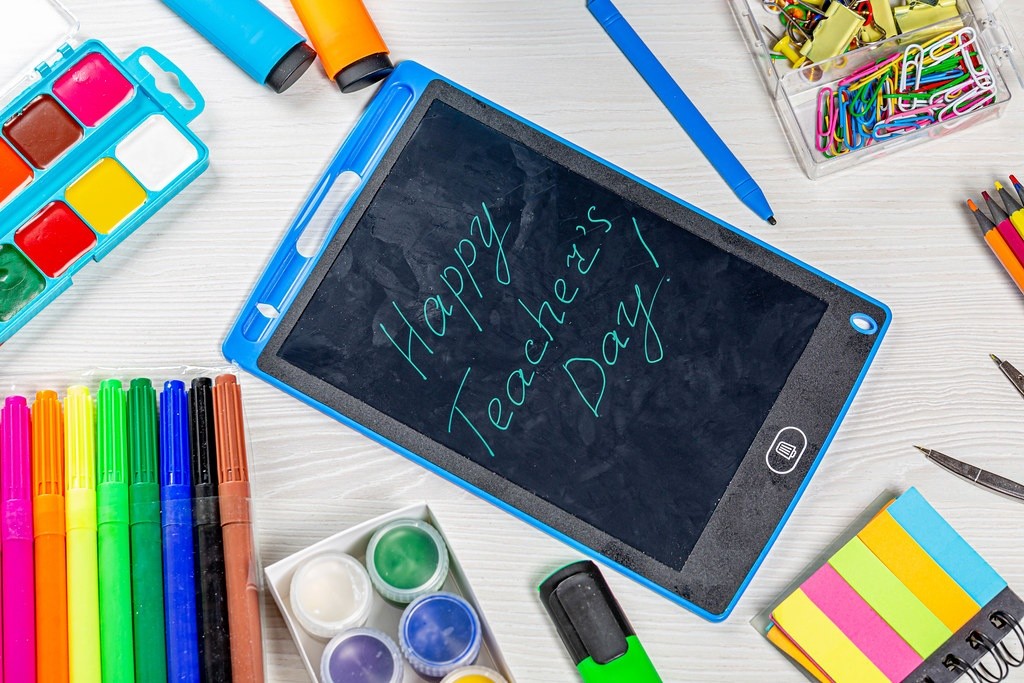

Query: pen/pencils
0;376;266;683
581;0;784;229
958;171;1024;298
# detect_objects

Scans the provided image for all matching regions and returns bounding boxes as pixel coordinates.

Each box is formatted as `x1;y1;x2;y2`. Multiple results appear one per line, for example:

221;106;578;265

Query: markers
536;558;663;683
160;0;395;94
